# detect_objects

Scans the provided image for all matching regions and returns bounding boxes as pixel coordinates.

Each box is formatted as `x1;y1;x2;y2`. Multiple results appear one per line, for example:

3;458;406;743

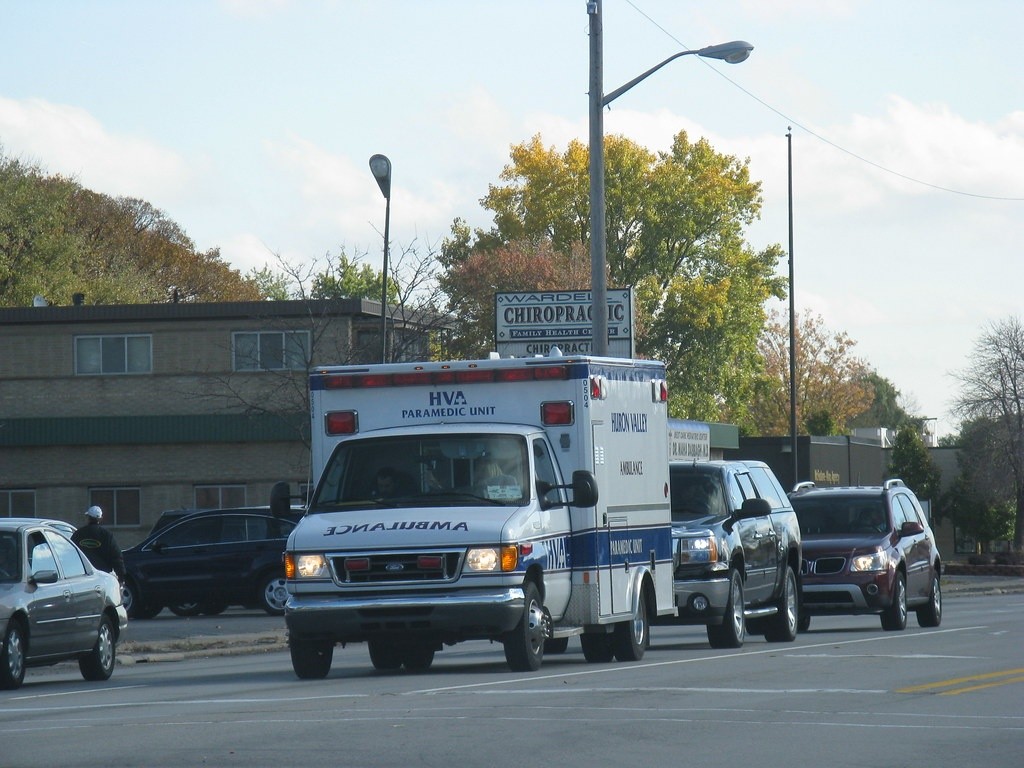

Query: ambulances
270;347;678;679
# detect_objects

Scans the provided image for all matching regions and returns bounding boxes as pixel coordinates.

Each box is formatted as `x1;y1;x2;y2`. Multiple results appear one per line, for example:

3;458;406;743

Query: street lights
368;153;392;364
588;0;755;357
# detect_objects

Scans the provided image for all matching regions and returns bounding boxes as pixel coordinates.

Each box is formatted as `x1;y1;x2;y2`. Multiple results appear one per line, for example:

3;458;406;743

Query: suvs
121;505;307;620
670;458;815;649
787;481;942;634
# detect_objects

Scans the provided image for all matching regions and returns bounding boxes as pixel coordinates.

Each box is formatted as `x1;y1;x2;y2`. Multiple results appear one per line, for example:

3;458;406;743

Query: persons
856;509;885;532
70;506;125;585
467;457;517;500
358;467;415;501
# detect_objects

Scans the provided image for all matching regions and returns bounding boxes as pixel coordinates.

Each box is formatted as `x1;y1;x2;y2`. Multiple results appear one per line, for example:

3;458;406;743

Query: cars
0;518;128;689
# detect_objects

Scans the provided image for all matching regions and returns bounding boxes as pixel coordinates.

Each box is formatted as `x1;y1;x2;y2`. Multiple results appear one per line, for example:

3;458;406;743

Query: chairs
369;470;417;501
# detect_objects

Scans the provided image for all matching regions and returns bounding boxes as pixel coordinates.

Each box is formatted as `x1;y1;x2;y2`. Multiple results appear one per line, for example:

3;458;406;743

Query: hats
84;505;103;519
